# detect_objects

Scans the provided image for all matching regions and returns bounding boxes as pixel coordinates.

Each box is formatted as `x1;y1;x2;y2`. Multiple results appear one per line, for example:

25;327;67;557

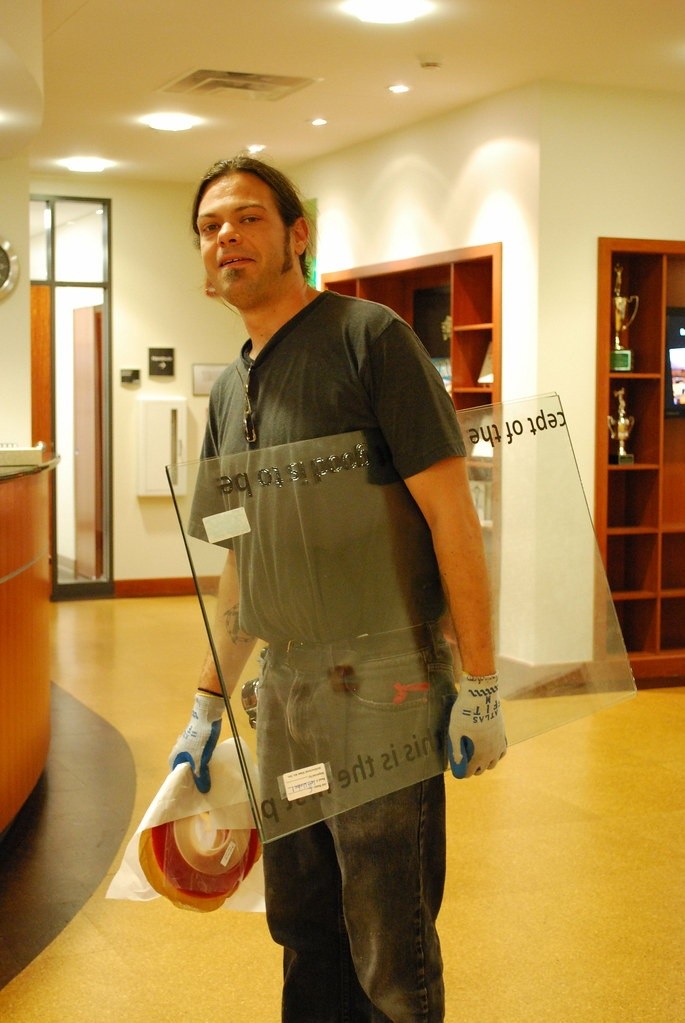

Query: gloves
169;693;226;794
448;671;507;778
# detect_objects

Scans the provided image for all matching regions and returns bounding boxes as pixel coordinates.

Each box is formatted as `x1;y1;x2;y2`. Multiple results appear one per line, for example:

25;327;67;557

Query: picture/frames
192;363;232;397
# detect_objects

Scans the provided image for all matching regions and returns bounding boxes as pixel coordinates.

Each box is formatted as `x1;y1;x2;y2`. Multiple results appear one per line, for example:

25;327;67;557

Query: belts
269;629;430;663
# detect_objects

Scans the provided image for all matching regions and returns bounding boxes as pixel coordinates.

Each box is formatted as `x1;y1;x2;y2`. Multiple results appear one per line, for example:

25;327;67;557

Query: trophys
605;387;636;465
611;260;640;372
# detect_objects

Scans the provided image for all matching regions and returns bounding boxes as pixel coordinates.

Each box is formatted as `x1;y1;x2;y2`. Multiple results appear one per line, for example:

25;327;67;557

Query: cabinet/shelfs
450;240;501;531
661;525;685;679
595;235;661;683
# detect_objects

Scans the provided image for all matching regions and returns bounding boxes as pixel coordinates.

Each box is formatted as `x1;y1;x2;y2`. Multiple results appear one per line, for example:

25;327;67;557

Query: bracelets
197;687;231;702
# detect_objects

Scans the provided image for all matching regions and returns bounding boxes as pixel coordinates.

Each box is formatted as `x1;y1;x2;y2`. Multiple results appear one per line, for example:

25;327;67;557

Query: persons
172;155;512;1020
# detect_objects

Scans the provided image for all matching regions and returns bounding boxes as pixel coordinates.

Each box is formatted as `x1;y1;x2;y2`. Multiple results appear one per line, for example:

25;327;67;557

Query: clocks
0;235;19;301
